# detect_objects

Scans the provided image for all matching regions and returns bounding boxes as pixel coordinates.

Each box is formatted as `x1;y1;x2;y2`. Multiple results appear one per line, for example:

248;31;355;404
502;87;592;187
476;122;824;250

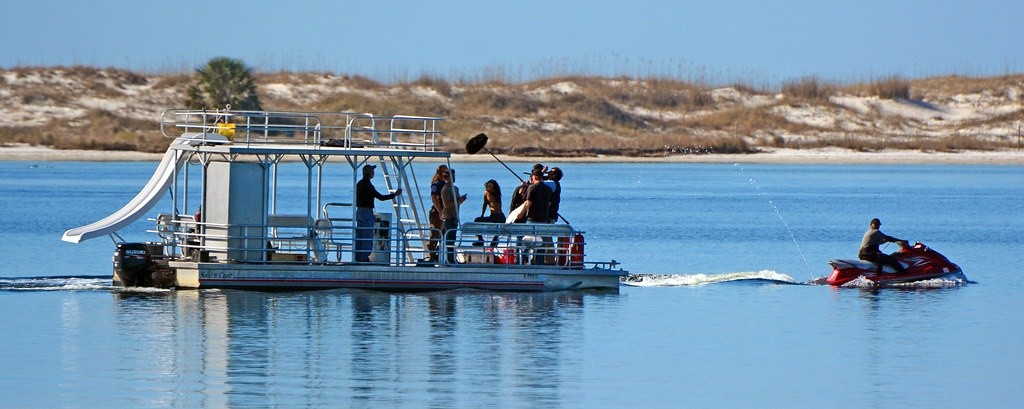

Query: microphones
465;133;488;155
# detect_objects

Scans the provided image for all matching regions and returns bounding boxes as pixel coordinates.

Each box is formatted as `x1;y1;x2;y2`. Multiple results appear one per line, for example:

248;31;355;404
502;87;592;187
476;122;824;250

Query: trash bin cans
368;212;392;265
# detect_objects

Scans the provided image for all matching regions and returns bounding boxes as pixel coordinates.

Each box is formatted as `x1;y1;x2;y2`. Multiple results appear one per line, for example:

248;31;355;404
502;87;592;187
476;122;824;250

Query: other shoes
490;240;499;246
473;239;484;246
356;259;375;265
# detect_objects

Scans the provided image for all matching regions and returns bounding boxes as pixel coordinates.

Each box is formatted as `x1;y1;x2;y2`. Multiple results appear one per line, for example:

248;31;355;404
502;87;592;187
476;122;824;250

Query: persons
858;219;909;274
472;179;506;246
355;164;402;262
426;165;467;263
509;164;562;263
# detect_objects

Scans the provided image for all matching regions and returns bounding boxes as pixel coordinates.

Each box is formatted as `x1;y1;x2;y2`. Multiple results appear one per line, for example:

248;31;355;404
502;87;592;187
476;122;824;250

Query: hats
362;164;376;172
871;218;881;225
532;164;543;168
533;169;543;179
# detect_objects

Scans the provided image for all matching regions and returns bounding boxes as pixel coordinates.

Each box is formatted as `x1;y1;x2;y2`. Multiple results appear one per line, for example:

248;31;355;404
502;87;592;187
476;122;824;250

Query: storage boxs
457;249;494;264
493;245;514;264
268;253;307;261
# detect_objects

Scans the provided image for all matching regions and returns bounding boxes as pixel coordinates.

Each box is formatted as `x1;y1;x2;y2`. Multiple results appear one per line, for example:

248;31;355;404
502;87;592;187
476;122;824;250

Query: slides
60;130;234;244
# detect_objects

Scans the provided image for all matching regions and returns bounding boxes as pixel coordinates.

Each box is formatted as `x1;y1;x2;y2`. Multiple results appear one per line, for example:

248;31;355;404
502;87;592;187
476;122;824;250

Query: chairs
313;218;342;263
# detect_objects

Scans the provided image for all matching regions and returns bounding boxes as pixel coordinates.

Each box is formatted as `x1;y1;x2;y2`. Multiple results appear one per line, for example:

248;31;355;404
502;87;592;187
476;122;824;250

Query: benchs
157;214;315;259
454;222;575;267
